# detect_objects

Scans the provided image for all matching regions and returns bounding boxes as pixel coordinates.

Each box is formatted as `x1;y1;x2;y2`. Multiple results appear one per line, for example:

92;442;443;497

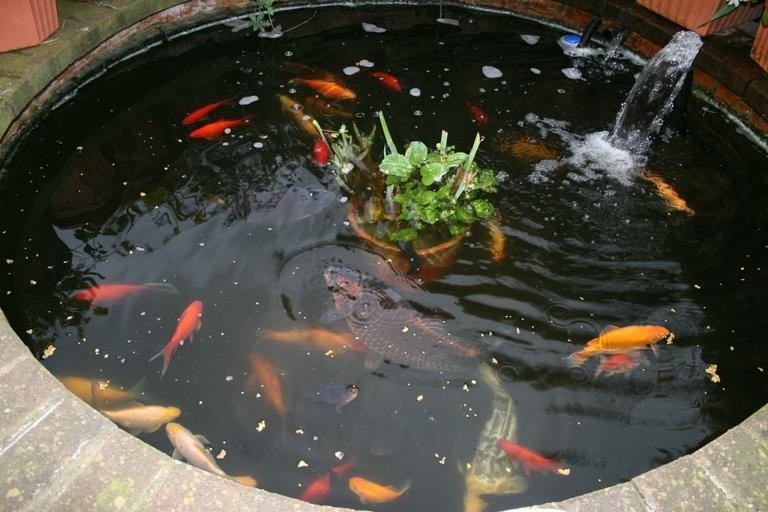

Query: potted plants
311;109;497;289
